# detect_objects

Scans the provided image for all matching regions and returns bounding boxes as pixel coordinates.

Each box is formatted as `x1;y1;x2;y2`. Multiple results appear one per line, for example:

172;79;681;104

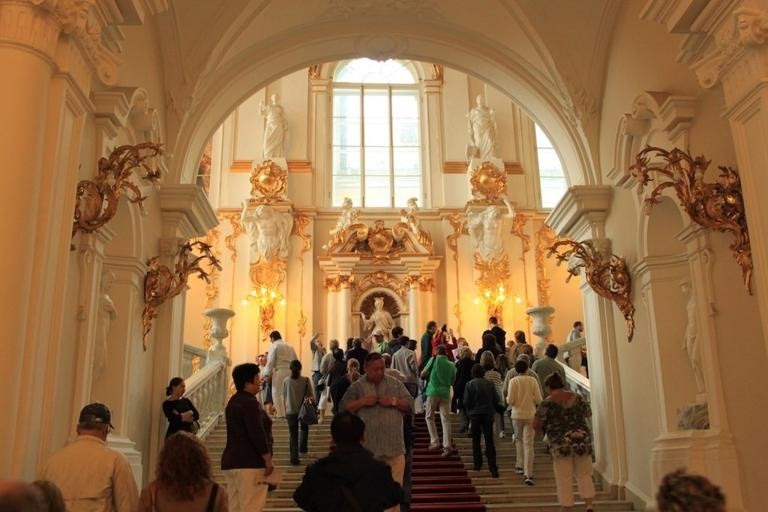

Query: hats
78;403;116;431
373;329;384;337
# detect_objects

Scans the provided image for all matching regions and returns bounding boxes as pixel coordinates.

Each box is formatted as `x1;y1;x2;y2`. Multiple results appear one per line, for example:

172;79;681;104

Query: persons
362;297;394;336
259;316;596;512
41;402;140;512
31;480;65;512
162;377;200;438
678;280;705;392
0;480;35;512
219;363;274;512
259;93;287;159
92;271;118;402
656;471;726;512
137;430;229;512
466;95;496;159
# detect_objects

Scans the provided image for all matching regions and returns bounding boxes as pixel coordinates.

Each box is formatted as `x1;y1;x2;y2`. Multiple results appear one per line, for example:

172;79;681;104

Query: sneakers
428;419;537;487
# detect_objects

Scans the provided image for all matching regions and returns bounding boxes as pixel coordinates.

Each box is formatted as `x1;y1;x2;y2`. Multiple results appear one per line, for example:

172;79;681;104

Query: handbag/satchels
421;387;427;401
317;375;326;391
297;396;318;426
